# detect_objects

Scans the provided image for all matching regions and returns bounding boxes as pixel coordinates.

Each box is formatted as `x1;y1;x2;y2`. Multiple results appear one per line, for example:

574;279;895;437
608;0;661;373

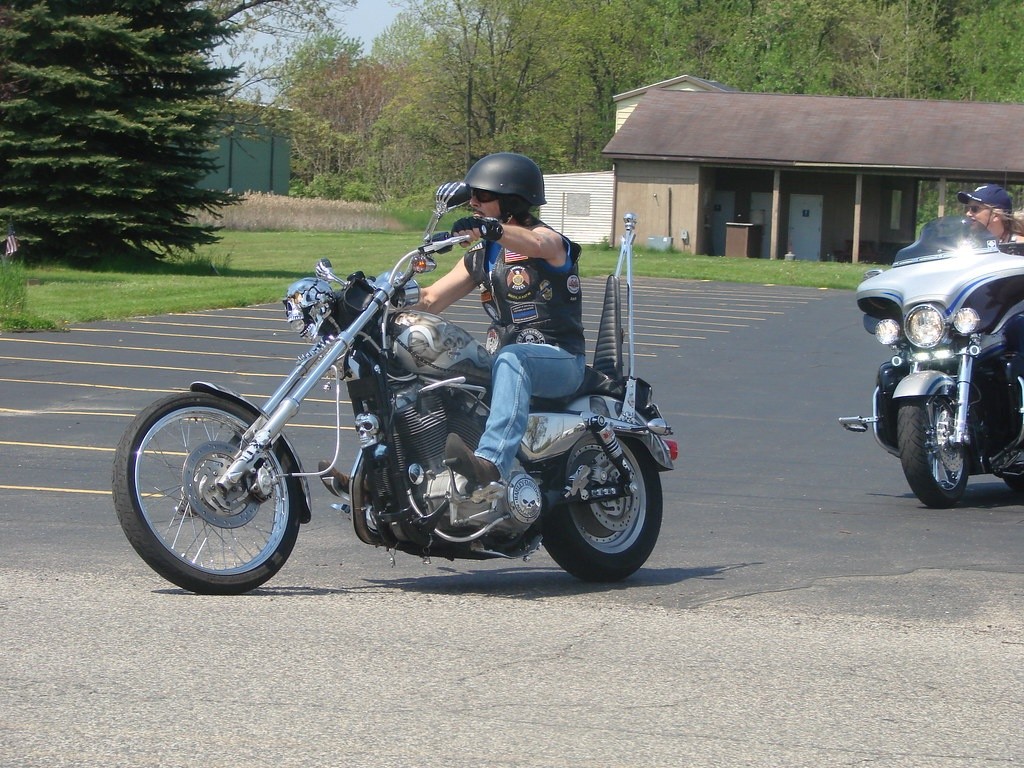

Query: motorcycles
110;181;679;595
838;213;1024;512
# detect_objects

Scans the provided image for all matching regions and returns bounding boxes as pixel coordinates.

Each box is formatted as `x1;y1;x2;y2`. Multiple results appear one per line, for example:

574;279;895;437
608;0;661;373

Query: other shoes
446;433;499;488
318;459;369;498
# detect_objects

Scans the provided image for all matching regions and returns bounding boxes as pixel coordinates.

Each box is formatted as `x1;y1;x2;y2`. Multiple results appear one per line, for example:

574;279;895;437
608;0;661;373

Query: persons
319;154;583;504
957;183;1024;244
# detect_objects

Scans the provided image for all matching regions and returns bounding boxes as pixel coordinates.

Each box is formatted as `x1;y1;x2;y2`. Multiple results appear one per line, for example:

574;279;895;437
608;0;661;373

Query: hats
958;184;1012;217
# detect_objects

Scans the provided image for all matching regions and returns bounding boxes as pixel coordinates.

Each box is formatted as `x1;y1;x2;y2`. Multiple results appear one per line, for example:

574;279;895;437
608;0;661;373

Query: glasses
470;188;503;204
962;204;990;214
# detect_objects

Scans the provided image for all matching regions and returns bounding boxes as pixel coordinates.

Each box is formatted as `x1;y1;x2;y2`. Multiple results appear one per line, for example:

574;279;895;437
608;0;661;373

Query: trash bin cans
726;223;763;259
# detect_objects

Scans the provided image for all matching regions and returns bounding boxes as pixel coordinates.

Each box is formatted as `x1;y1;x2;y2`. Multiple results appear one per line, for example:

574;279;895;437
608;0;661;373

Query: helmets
463;153;547;206
